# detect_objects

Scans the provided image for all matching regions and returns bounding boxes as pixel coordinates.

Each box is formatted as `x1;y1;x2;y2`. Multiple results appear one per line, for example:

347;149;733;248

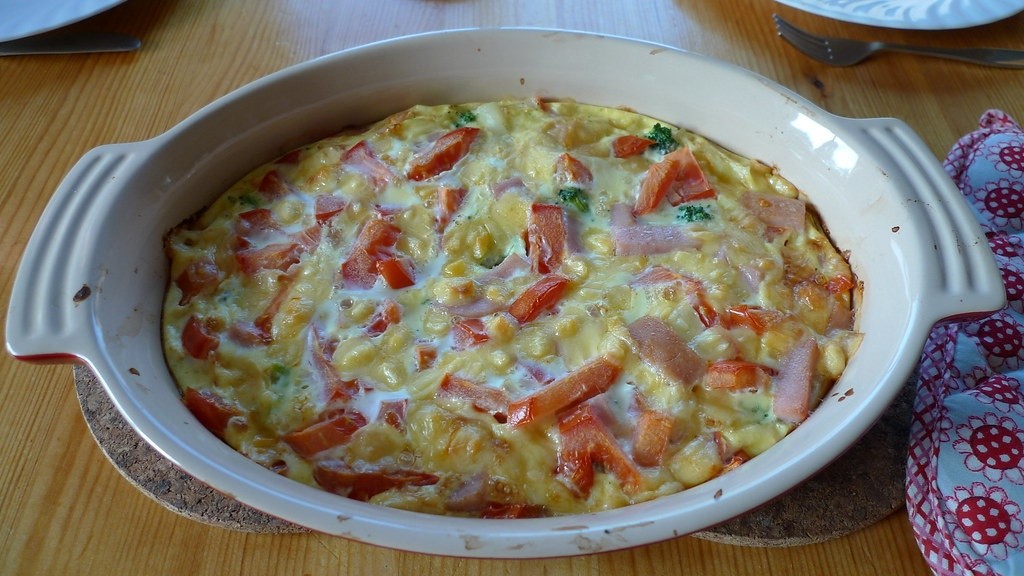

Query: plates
774;0;1024;30
0;0;127;47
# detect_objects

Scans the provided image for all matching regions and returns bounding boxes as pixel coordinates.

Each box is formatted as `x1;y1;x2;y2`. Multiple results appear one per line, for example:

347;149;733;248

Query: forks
772;14;1024;77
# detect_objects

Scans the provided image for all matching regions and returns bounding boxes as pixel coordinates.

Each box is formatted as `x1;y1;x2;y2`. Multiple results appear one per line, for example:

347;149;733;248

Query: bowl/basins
6;27;1007;560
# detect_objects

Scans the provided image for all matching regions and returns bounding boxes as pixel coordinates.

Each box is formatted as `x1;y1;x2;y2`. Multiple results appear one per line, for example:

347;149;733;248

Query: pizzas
161;98;855;519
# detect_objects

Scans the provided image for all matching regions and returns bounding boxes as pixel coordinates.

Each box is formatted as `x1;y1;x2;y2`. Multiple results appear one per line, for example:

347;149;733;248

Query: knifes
1;35;140;55
